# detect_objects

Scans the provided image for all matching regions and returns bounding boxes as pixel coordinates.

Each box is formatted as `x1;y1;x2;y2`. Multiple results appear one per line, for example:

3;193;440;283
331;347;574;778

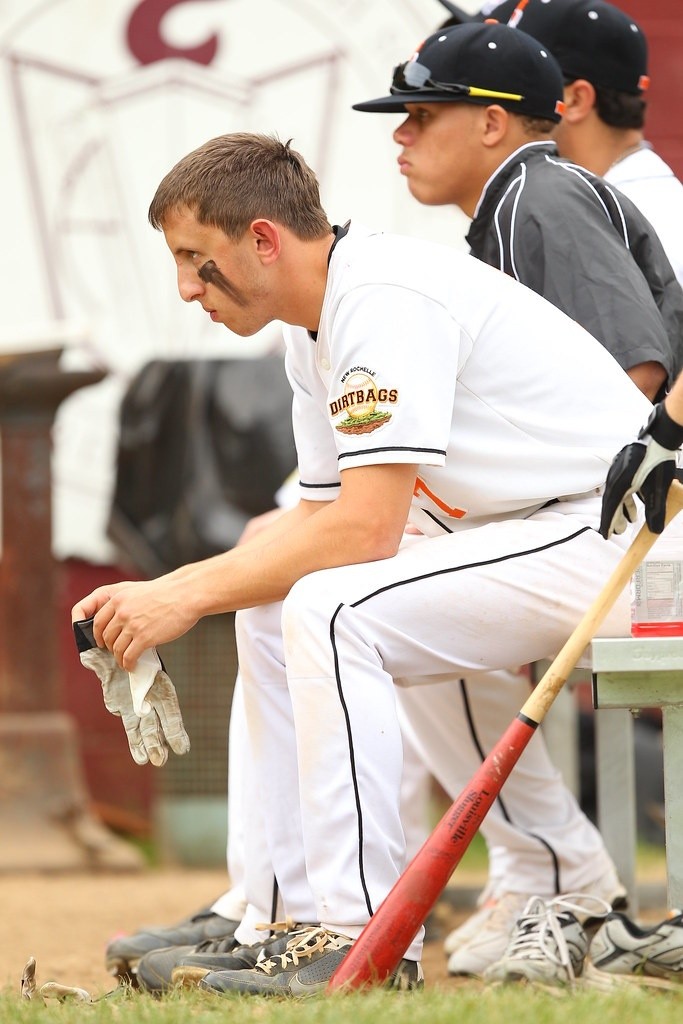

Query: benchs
532;638;683;921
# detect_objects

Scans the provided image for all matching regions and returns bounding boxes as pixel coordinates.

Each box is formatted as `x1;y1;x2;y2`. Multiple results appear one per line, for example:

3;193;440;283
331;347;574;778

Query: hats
353;15;567;122
440;0;647;94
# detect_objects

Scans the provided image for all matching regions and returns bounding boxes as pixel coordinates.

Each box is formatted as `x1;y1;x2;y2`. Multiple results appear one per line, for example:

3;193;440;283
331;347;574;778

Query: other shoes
445;892;494;954
449;896;520;977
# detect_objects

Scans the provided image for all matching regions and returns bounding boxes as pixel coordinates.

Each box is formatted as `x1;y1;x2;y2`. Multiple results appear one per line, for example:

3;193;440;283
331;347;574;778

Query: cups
630;535;683;637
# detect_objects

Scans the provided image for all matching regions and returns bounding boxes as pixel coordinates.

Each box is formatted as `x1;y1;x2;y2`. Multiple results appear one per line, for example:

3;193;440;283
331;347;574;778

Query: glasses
387;64;528;101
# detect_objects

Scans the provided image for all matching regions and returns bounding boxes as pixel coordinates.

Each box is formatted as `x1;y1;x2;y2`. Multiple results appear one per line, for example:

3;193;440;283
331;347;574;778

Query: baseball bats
324;481;683;999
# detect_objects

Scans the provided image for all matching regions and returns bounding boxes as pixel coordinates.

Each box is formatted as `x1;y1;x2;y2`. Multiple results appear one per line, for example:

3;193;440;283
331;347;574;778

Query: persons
72;136;646;999
232;0;682;981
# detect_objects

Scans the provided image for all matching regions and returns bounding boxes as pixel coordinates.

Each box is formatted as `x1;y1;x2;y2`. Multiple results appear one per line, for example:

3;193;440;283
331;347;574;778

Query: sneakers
135;931;245;995
198;929;425;1000
107;910;245;982
172;918;310;986
497;891;611;994
580;911;682;990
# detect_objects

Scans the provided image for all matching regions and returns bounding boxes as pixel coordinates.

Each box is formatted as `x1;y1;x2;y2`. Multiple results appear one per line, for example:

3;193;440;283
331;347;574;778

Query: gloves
73;617;193;766
599;402;682;539
